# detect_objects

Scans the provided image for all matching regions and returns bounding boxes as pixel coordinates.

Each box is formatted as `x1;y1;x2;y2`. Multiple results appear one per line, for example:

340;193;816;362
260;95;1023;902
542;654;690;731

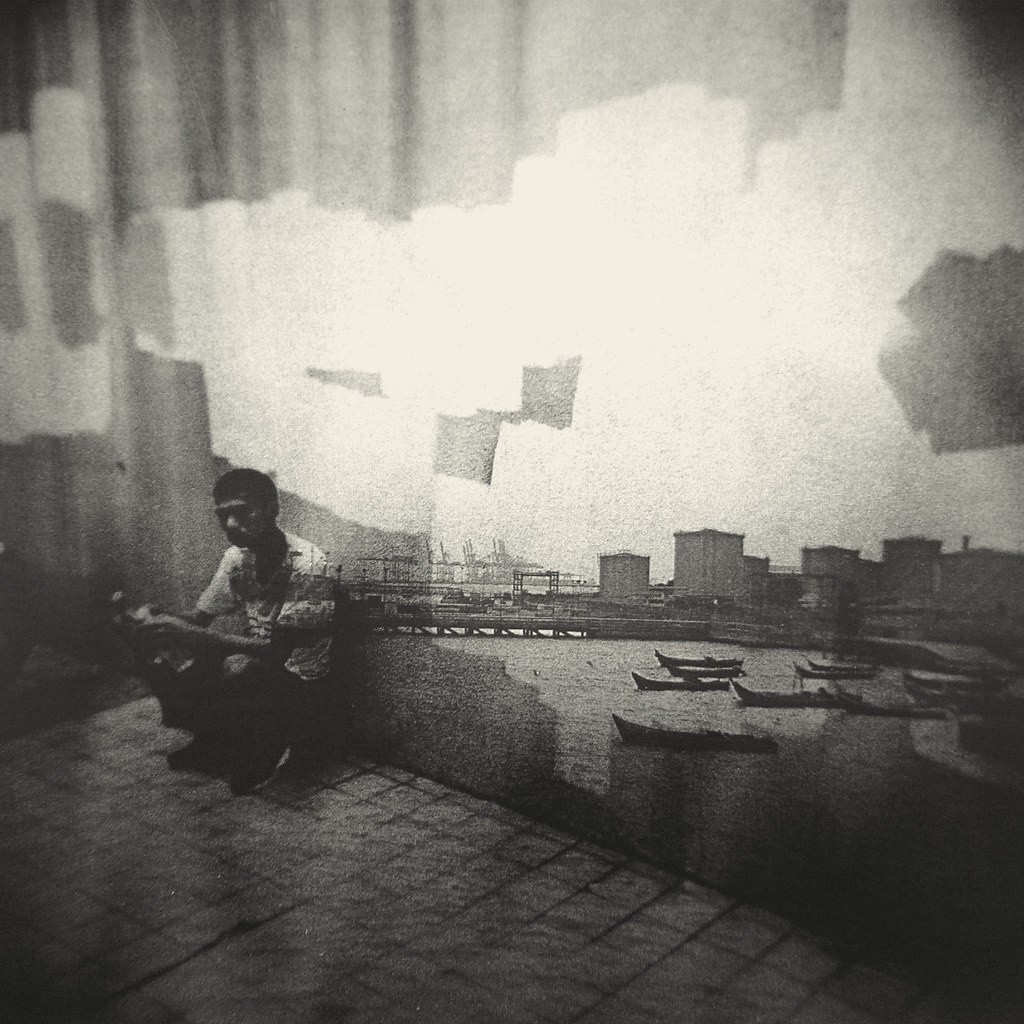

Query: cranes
355;536;542;583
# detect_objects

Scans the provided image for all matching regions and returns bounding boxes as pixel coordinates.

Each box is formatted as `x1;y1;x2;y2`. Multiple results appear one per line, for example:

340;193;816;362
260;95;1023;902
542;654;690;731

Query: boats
833;681;946;720
792;660;863;680
902;678;975;704
807;659;863;672
612;713;778;752
666;663;745;678
655;648;744;668
730;678;862;707
632;671;730;691
901;672;973;690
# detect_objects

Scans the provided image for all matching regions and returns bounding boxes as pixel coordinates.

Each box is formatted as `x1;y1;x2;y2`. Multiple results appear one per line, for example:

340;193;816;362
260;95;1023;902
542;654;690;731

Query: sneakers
227;722;295;797
163;736;236;770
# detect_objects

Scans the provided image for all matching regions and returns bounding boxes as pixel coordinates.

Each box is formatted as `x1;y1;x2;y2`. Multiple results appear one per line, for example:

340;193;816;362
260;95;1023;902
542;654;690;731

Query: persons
113;468;342;794
